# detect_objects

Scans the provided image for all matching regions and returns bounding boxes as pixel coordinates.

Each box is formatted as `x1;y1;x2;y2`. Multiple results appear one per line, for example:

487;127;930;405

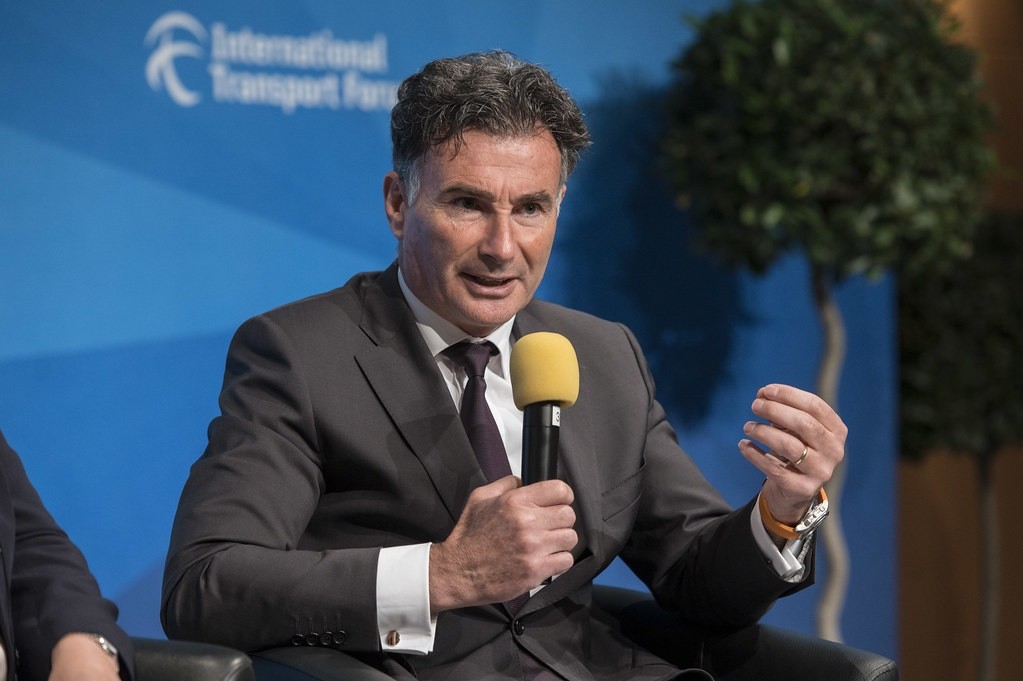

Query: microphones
510;331;580;586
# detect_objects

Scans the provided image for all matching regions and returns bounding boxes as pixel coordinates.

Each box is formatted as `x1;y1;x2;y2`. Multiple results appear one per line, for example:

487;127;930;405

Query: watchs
759;486;830;541
93;633;118;656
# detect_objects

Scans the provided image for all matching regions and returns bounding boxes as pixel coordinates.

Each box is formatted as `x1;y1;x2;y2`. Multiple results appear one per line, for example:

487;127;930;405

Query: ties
439;342;565;681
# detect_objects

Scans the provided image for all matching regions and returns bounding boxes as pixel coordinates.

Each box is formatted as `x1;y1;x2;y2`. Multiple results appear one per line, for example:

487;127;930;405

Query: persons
159;49;848;681
0;432;136;681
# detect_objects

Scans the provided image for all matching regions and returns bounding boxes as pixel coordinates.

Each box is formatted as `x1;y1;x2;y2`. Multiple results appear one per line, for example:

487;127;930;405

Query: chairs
125;584;897;681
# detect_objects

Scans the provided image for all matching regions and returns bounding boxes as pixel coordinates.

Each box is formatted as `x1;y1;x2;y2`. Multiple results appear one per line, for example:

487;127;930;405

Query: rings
790;444;808;466
